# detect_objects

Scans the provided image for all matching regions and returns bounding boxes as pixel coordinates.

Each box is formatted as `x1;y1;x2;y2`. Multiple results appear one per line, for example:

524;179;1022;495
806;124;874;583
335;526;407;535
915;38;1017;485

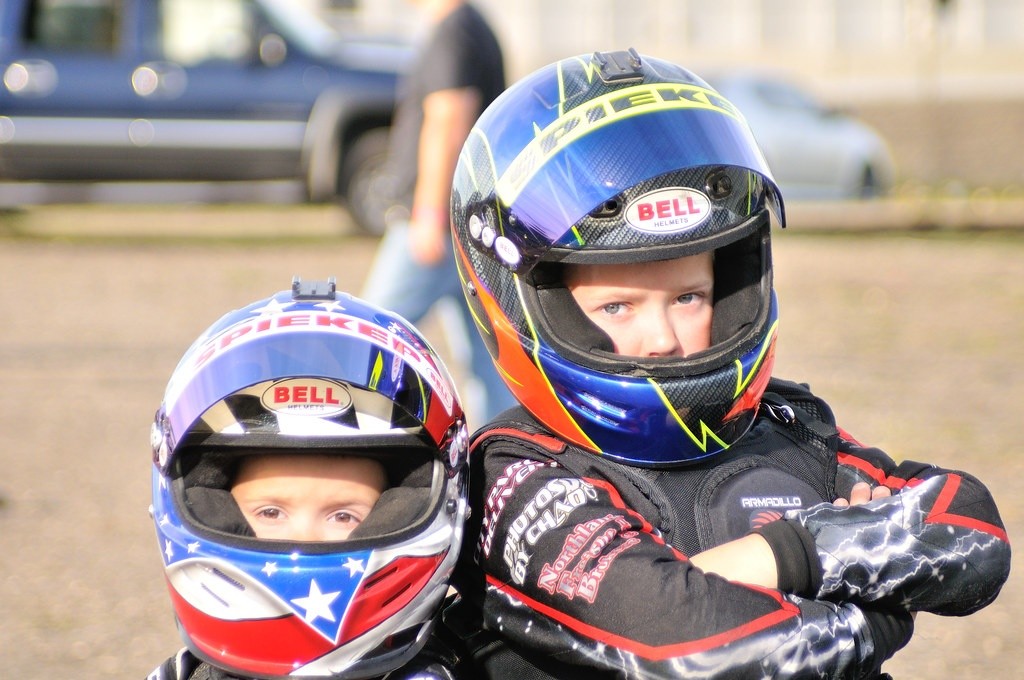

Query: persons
360;1;518;427
450;50;1010;679
145;276;470;680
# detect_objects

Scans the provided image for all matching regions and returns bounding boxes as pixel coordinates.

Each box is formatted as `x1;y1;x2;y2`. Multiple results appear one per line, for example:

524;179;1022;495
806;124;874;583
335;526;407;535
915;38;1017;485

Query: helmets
450;46;789;473
149;273;471;678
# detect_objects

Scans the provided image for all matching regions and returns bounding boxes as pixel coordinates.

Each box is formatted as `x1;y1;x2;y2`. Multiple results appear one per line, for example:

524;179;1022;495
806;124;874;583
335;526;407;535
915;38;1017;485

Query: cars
0;0;430;237
